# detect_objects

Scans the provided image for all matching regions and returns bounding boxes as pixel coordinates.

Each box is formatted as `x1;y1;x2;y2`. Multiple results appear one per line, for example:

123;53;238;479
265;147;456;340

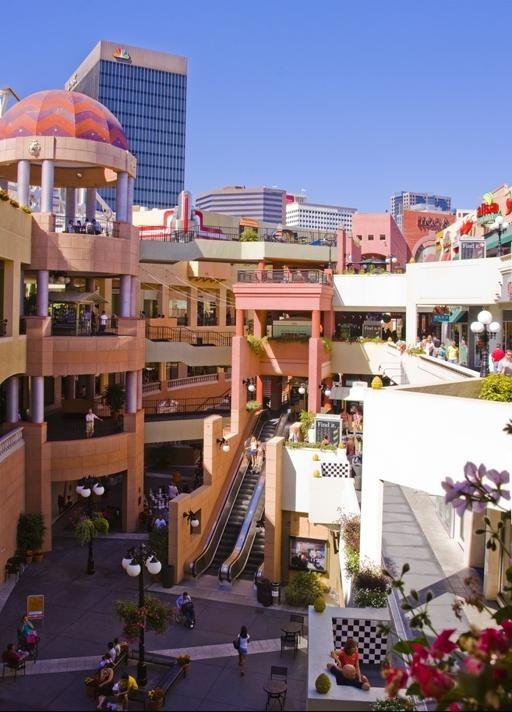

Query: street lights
76;474;105;576
122;543;162;686
490;216;508;257
470;305;502;377
385;254;397;273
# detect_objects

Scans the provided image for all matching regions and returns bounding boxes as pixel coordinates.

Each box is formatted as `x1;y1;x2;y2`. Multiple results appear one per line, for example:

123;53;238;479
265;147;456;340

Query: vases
145;698;163;712
182;662;193;680
84;686;97;698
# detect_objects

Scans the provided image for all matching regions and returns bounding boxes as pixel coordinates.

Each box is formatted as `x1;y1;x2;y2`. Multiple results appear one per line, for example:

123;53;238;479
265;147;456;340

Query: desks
262;680;287;712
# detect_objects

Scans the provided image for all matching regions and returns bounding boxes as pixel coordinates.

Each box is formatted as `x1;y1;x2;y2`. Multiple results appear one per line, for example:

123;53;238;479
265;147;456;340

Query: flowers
84;675;94;686
176;654;191;664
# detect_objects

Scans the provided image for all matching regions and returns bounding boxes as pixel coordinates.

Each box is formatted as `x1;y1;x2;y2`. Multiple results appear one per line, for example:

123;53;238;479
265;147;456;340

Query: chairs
66;224;103;235
2;632;40;683
262;614;305;706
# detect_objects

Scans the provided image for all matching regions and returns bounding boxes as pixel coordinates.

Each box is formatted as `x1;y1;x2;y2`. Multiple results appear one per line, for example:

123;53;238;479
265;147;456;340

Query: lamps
254;517;265;534
217;362;409;452
342;312;369;320
183;508;199;528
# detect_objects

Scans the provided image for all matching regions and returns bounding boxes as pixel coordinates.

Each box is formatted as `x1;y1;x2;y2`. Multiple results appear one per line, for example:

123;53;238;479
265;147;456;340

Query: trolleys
175;602;196;629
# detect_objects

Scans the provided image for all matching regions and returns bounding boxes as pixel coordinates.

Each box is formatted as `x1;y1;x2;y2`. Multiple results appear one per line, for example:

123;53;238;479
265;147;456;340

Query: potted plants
14;511;48;565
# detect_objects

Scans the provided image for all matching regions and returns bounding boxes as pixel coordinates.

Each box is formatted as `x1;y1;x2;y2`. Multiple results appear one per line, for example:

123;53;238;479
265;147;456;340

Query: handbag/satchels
232;636;242;650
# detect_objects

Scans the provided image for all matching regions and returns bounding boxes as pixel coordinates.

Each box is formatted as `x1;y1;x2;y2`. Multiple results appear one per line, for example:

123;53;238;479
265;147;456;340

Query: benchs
94;650;186;707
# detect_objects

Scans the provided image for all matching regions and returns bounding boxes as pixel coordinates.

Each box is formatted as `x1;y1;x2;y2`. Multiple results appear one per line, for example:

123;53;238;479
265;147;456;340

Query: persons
497;348;512;374
17;614;39;647
153;513;166;530
242;436;266;473
67;217;103;235
488;346;504;372
416;333;459;363
176;591;192;624
330;638;368;684
98;310;108;334
235;625;250;675
6;642;31;663
321;433;330;446
85;408;104;439
85;636;139;711
326;661;369;690
166;481;179;500
